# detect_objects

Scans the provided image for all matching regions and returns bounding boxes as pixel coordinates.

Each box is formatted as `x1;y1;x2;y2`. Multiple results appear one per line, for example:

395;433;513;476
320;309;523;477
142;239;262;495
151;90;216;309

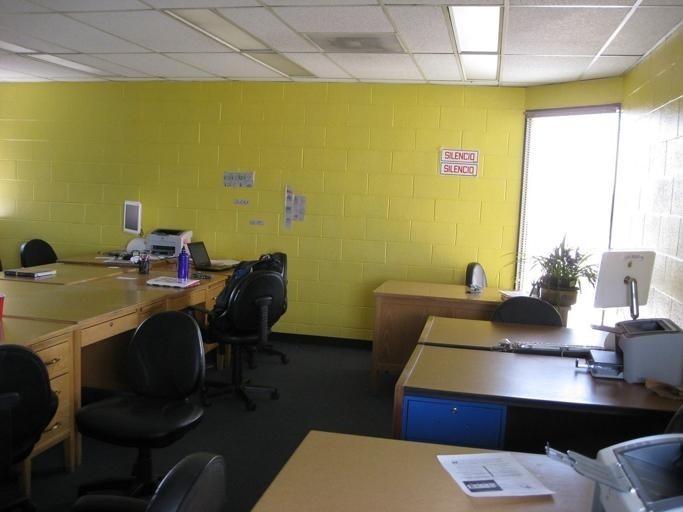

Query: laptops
187;240;234;272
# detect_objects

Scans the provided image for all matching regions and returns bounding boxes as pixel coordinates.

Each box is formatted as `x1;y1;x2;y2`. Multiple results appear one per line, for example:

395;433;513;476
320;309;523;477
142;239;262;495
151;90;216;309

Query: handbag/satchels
208;254;281;320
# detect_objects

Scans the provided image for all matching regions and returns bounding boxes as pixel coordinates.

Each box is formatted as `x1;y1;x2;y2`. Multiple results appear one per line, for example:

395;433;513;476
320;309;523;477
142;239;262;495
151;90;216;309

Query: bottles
178;245;189;278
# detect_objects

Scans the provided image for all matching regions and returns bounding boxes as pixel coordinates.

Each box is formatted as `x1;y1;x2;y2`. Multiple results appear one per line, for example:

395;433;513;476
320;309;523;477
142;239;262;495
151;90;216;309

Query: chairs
73;310;205;497
72;453;229;512
20;239;58;268
0;345;58;512
465;262;487;288
243;252;291;369
491;296;563;327
187;271;286;410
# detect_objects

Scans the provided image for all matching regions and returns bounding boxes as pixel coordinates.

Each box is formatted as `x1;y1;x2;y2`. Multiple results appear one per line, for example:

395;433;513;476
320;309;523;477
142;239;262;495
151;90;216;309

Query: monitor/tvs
592;250;656;310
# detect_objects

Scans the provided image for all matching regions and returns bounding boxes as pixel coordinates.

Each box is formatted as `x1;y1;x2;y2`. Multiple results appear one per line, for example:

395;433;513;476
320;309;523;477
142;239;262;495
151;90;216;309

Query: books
4;268;56;278
146;275;200;289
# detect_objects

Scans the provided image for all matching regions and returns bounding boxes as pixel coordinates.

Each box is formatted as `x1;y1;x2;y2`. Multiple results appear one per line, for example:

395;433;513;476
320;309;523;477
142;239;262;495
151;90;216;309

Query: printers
574;315;682;383
543;432;683;512
145;229;193;257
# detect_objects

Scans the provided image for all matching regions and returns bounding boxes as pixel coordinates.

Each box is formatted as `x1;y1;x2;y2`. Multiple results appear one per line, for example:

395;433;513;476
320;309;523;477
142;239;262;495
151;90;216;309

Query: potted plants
500;232;597;306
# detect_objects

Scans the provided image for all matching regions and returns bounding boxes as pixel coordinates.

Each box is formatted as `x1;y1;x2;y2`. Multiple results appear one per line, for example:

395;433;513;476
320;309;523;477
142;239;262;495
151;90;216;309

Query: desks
417;315;615;357
56;251;177;266
372;279;571;368
0;279;166;465
250;430;596;512
391;343;683;448
148;257;237;368
0;316;78;500
69;269;228;370
0;261;141;285
